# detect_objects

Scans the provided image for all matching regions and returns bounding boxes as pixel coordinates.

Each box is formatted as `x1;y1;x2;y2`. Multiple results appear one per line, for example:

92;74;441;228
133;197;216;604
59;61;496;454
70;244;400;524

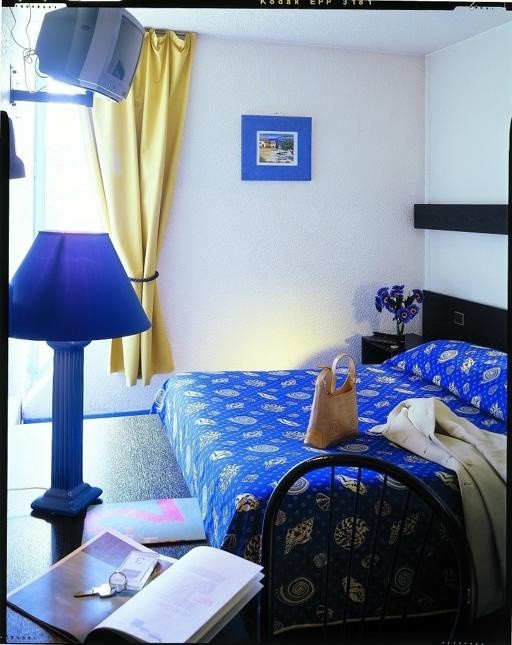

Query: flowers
374;283;426;332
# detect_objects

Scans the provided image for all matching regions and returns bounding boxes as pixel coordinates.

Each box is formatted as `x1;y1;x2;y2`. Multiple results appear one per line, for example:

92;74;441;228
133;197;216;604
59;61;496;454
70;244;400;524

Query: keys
74;569;131;599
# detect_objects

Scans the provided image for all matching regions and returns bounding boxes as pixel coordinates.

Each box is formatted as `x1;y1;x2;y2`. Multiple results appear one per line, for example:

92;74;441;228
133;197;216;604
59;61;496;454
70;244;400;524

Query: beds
164;290;512;633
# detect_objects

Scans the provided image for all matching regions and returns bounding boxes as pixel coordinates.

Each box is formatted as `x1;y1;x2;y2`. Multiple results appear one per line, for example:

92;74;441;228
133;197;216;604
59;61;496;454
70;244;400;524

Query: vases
396;322;404;337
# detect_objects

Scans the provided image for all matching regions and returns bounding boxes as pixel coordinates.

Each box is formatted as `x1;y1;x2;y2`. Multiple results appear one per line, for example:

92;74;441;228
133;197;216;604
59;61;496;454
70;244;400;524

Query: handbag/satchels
303;352;359;450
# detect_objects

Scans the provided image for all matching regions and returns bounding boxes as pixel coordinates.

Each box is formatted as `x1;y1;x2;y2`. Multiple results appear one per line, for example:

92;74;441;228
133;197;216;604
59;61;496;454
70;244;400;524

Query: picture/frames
242;115;311;181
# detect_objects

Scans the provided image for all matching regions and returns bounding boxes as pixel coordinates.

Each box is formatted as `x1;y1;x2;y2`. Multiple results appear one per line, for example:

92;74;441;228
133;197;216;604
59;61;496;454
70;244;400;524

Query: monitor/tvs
34;7;145;103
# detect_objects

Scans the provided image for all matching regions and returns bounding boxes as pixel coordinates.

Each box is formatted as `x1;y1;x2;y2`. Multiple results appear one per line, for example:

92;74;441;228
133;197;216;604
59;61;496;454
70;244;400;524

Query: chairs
260;453;476;645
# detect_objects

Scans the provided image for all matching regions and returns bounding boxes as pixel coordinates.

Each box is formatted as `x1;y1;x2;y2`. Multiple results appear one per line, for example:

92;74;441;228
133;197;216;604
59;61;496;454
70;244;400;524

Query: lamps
9;231;154;515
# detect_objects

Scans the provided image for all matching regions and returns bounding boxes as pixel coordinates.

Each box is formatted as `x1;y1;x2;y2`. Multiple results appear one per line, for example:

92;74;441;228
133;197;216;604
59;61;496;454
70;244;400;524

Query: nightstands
362;333;426;364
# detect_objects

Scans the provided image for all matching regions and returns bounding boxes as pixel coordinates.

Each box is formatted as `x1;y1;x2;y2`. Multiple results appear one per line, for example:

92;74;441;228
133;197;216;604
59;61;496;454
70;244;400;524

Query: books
81;496;208;550
7;528;267;642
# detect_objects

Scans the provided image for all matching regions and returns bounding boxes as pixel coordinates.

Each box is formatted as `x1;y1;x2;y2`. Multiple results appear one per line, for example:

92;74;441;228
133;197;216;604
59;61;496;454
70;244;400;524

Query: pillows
382;334;507;421
